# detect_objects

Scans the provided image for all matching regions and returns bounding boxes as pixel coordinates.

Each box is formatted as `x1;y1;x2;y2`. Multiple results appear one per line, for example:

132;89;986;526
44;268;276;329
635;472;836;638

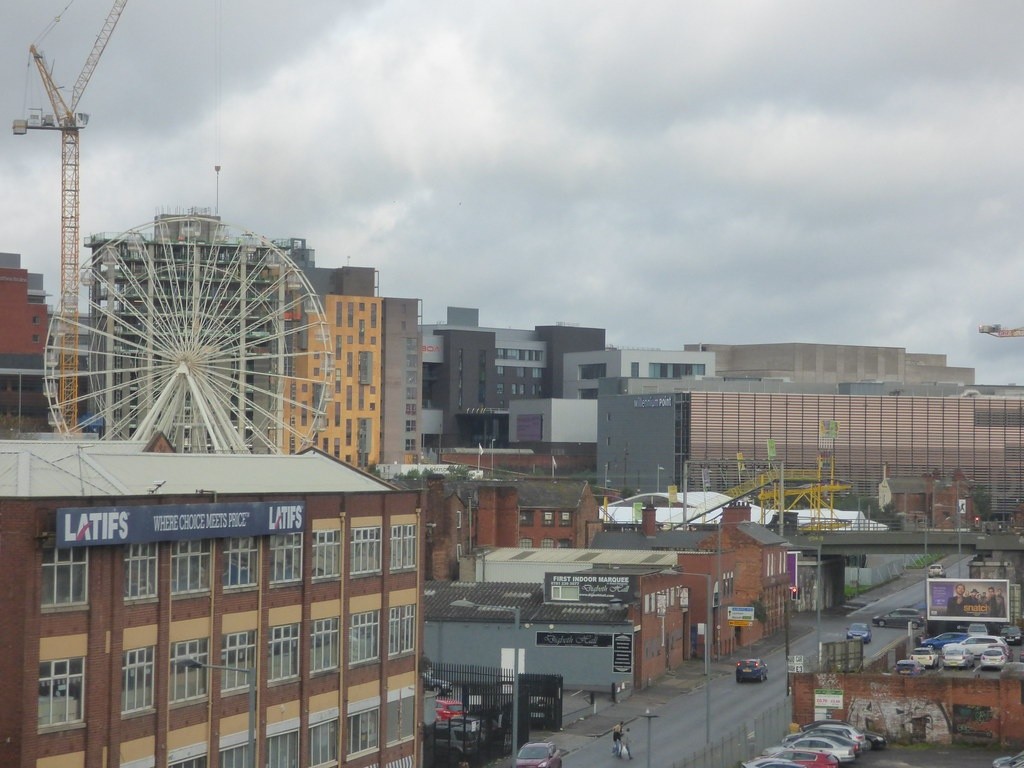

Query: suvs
514;741;562;768
929;563;947;578
874;607;923;630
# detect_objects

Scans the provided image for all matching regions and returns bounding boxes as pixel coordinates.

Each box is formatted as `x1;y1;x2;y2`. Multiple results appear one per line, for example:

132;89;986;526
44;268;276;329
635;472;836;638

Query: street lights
973;517;980;527
450;599;520;760
887;477;908;519
660;570;710;744
782;542;821;664
896;512;928;609
932;479;974;578
717;520;754;660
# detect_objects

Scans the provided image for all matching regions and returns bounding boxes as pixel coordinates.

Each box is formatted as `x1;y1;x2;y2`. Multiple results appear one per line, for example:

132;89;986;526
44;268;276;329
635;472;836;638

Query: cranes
14;0;125;430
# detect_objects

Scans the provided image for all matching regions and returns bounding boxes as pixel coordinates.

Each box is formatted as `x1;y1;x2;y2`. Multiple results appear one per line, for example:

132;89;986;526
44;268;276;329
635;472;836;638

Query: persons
946;584;1005;616
611;721;634;760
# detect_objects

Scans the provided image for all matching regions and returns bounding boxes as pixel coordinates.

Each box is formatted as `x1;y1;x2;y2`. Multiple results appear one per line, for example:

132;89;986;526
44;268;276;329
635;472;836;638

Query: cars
420;673;453;695
847;622;872;644
892;622;1024;678
736;658;767;683
435;701;464;720
991;750;1024;768
739;719;887;768
